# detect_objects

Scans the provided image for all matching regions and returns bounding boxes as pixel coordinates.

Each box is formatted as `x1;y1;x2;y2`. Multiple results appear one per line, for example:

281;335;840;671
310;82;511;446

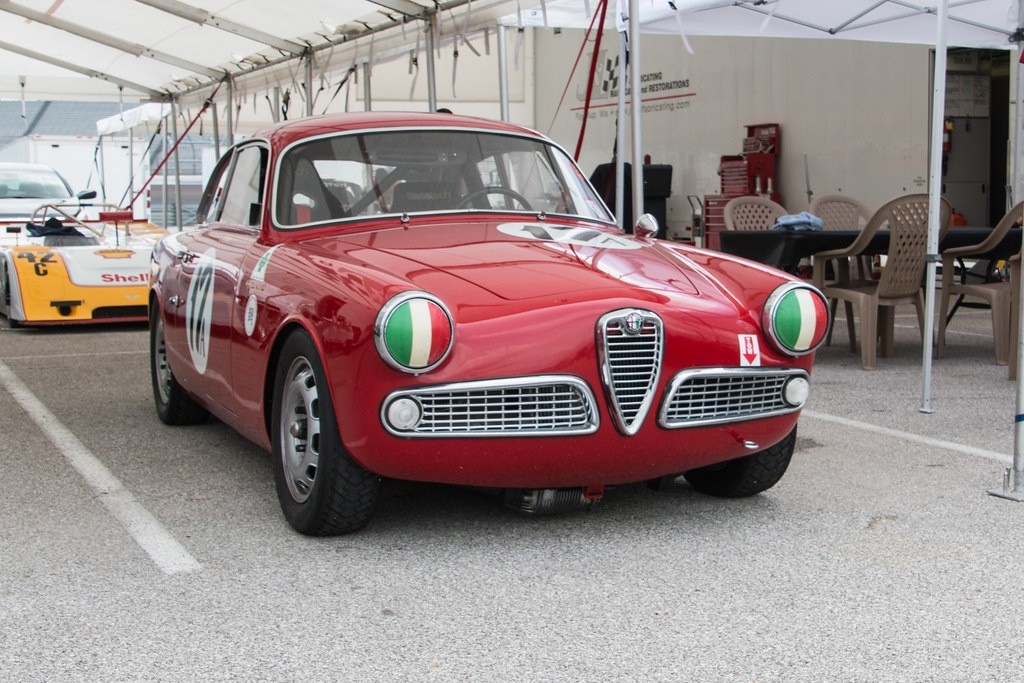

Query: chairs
724;193;793;230
934;200;1024;379
810;190;884;352
813;192;954;369
271;151;477;225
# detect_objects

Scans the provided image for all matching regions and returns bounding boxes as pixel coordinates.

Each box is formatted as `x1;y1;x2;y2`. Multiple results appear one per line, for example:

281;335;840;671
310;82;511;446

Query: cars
0;202;172;329
148;109;833;538
0;162;96;221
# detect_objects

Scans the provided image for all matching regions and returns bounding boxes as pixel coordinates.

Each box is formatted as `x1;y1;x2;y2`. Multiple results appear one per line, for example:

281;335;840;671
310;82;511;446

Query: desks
715;224;1023;293
